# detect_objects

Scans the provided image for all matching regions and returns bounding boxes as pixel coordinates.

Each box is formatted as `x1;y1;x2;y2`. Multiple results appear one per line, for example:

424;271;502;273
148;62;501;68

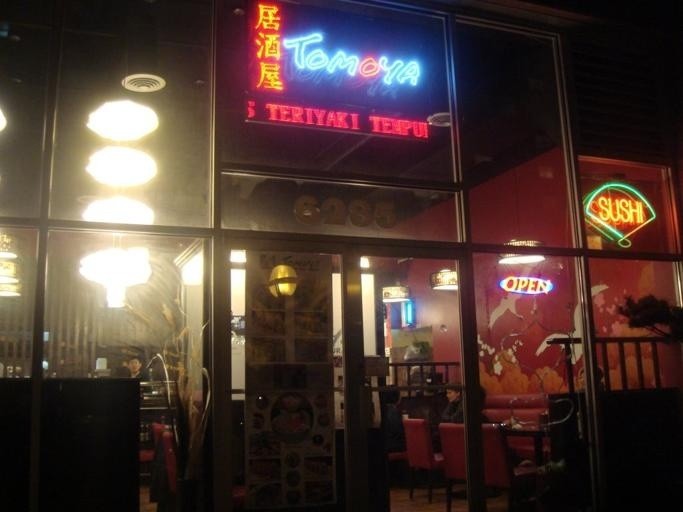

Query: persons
123;357;144;380
380;387;407;452
440;384;464;422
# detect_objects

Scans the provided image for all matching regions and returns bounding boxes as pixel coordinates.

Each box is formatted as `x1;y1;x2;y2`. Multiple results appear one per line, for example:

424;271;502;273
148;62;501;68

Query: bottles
141;421;150;444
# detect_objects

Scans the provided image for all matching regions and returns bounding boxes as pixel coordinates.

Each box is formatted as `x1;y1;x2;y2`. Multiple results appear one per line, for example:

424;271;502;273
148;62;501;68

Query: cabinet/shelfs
243;245;337;509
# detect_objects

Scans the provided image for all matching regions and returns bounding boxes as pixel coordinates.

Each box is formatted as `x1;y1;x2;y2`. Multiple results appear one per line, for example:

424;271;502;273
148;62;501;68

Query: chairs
379;392;553;503
